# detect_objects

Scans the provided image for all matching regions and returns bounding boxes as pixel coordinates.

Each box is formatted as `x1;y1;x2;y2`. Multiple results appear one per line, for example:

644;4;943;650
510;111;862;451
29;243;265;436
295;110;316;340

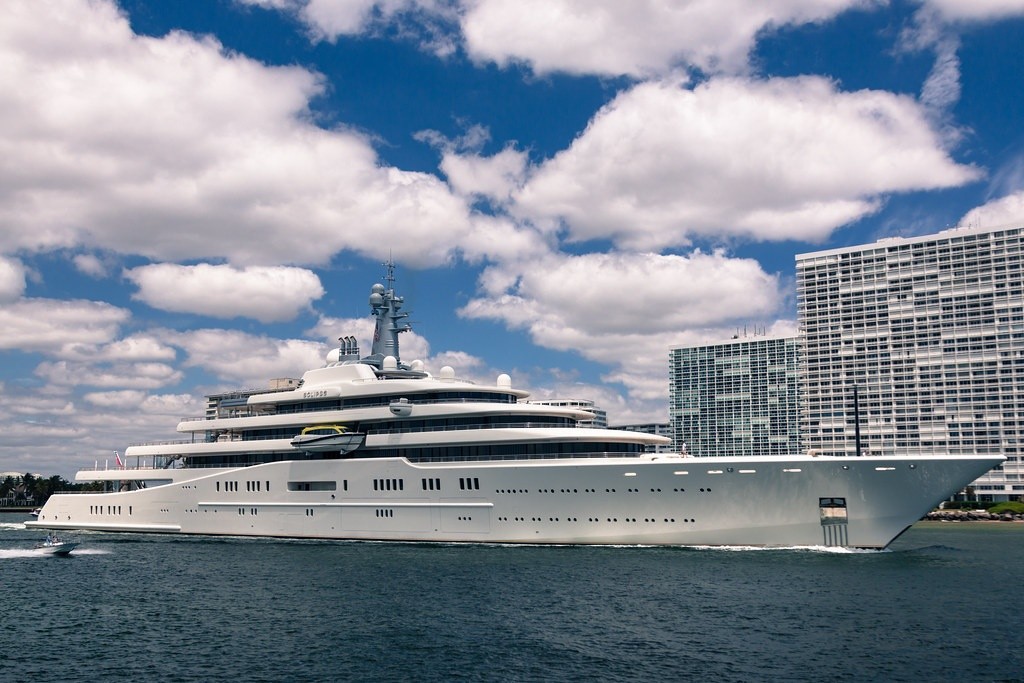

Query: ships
24;249;1008;550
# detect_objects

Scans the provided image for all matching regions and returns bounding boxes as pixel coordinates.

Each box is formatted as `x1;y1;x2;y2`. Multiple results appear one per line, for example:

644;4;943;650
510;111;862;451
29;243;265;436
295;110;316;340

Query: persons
682;441;688;458
863;450;872;456
46;532;58;547
807;450;815;455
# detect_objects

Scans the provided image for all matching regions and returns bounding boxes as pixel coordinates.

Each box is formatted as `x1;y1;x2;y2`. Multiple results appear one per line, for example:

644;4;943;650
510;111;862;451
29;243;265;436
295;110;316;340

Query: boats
28;508;42;517
31;540;81;556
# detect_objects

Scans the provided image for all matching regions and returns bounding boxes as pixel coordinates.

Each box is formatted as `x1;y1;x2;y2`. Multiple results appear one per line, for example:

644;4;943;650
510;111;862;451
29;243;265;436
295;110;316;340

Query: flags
115;452;122;465
